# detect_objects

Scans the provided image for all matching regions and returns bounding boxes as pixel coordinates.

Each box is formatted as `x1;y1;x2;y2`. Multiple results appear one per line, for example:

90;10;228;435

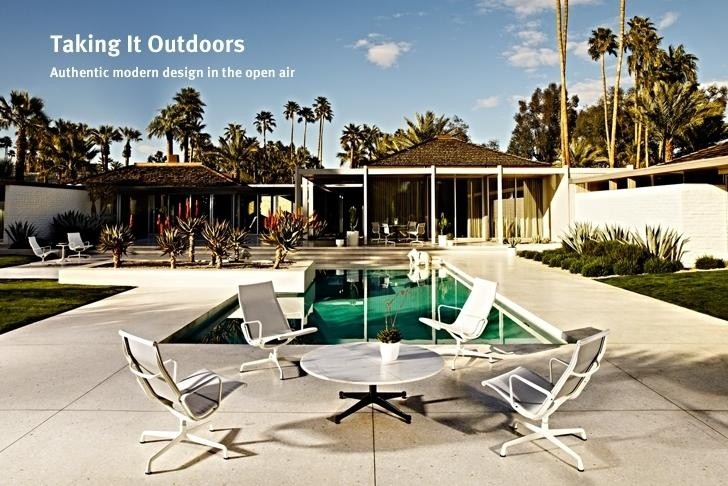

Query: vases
336;239;344;247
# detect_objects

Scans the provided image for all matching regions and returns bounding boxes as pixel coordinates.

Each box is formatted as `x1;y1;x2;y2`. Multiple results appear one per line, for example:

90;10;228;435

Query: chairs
27;232;94;266
481;328;611;473
370;218;426;246
418;278;500;371
236;280;319;379
117;328;247;476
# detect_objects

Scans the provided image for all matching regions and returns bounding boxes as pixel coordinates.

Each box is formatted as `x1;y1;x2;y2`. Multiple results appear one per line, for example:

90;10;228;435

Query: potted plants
346;206;359;246
438;217;448;247
377;327;403;364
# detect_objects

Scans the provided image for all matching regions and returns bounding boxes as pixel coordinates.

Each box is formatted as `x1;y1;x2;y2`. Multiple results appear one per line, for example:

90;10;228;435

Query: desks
301;342;444;424
